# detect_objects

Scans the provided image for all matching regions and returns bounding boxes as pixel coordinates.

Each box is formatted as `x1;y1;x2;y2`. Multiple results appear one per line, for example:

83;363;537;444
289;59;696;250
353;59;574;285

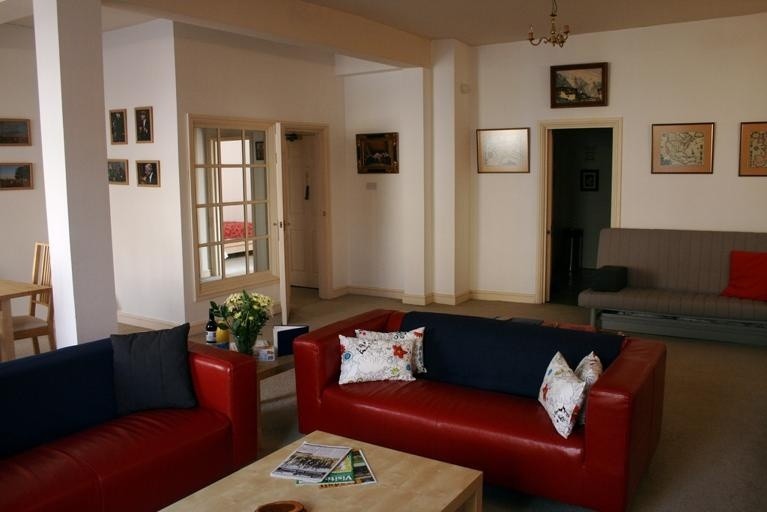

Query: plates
254;500;304;512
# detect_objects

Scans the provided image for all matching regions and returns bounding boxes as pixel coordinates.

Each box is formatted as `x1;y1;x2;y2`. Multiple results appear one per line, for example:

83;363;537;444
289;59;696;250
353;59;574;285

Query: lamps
527;0;571;48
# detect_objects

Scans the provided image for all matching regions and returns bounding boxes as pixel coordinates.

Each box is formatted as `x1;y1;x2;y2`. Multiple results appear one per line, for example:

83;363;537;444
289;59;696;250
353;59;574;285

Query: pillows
573;351;603;425
355;326;427;373
109;322;198;410
538;350;586;439
721;248;767;301
338;334;416;385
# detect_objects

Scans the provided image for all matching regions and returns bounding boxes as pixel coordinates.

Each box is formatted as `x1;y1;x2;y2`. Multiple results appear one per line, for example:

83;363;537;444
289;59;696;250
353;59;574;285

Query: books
273;325;310;356
296;450;354;484
319;450;376;489
271;441;352;482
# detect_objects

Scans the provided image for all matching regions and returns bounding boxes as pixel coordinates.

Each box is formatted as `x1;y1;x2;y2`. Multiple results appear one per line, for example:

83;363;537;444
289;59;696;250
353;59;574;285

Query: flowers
209;288;273;336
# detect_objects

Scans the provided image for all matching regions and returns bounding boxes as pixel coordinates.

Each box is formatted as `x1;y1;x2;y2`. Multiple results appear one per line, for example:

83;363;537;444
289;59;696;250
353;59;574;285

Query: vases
234;336;257;356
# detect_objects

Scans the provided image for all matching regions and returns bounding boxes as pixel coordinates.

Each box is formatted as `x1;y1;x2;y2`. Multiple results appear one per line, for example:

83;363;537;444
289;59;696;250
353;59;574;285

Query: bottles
205;309;216;346
216;316;229;350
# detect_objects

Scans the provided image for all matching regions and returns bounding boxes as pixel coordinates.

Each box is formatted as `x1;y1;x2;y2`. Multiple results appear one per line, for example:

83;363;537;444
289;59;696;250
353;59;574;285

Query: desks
0;279;52;362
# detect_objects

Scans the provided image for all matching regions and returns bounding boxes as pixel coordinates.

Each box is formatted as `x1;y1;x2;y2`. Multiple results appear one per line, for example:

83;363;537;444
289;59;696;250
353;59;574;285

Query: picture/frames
135;106;154;144
109;108;128;144
738;122;767;177
135;160;161;187
0;162;34;190
107;159;130;185
475;127;531;174
255;141;265;160
550;61;609;109
355;132;400;174
650;122;715;174
0;118;32;147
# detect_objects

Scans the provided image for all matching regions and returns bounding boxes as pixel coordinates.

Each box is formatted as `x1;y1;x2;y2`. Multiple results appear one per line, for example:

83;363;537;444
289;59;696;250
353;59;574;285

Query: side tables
189;324;295;450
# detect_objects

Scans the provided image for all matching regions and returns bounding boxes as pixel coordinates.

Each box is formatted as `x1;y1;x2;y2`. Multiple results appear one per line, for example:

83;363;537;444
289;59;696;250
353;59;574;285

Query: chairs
12;242;57;354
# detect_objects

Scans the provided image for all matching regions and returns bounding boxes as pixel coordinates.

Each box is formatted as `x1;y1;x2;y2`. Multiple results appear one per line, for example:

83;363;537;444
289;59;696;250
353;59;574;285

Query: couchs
578;228;767;348
0;339;262;512
222;221;253;260
292;308;667;511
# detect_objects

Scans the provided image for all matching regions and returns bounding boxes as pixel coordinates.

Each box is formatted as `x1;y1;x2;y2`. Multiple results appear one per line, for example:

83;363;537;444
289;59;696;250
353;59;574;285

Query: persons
141;164;157;184
138;111;152;141
